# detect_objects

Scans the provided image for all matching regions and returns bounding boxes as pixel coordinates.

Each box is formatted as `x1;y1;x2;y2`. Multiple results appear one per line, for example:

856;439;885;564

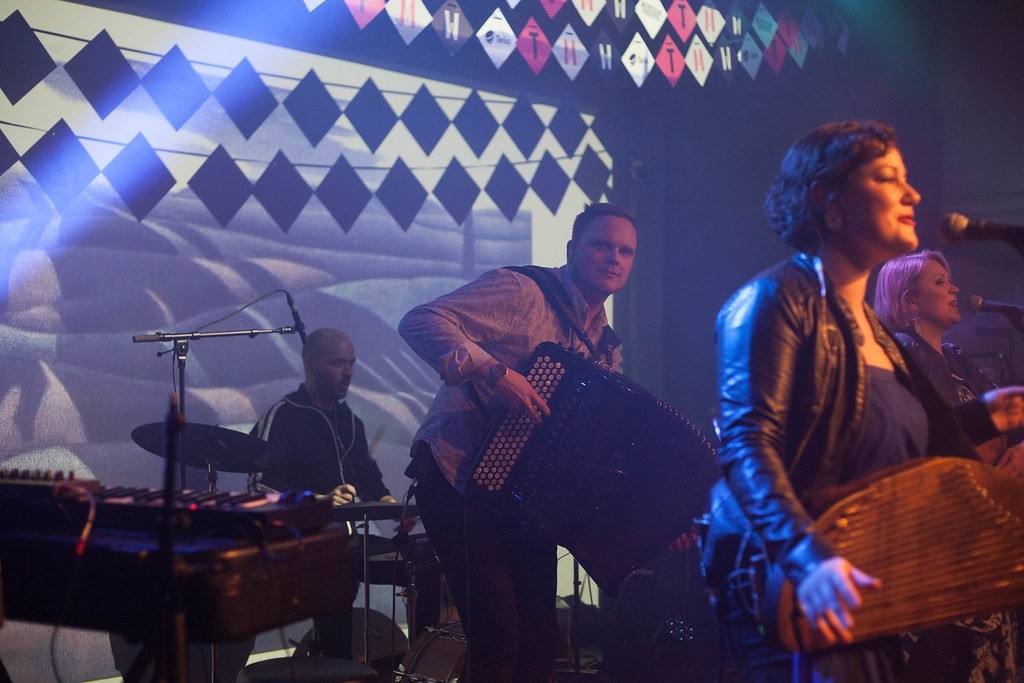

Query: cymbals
130;419;272;472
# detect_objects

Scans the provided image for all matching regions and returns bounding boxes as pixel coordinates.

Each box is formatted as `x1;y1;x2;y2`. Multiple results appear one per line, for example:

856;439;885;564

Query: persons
399;203;638;683
702;122;1024;683
872;249;1024;683
247;327;439;662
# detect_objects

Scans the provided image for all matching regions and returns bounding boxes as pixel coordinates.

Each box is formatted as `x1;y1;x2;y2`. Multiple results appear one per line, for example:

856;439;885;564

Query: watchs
485;362;508;390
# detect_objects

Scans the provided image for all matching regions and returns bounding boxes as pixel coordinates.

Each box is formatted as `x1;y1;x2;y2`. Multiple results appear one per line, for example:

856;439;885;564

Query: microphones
405;480;418;501
287;293;307;344
940;214;1024;243
966;295;1024;316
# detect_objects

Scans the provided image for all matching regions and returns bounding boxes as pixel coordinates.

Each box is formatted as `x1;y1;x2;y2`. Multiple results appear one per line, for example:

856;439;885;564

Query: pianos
0;464;364;683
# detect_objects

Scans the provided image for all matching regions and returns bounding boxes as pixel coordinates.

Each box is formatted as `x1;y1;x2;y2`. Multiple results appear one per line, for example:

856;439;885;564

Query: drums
400;619;468;682
299;607;406;682
239;654;379;682
